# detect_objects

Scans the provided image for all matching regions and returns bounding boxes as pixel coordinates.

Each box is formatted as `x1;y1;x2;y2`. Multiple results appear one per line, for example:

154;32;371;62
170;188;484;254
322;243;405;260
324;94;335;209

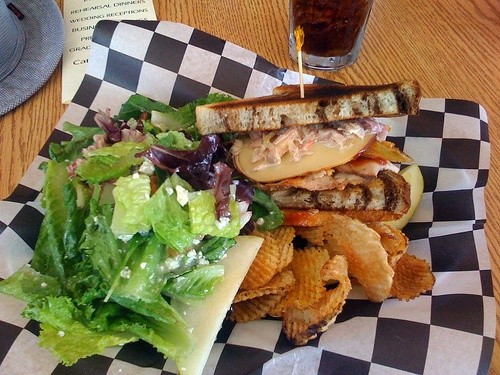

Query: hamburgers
200;79;424;214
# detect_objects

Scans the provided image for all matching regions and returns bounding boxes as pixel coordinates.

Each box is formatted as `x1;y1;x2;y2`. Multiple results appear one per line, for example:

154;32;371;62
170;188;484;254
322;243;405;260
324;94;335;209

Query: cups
288;0;374;71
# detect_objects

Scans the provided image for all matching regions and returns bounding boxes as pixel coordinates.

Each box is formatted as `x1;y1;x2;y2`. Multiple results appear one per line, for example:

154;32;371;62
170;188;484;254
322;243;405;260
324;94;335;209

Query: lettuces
1;93;284;375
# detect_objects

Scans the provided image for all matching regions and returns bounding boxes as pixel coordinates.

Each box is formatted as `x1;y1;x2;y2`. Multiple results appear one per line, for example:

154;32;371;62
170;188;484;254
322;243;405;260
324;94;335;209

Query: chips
228;216;438;343
